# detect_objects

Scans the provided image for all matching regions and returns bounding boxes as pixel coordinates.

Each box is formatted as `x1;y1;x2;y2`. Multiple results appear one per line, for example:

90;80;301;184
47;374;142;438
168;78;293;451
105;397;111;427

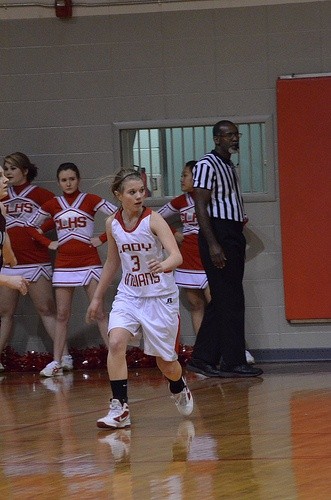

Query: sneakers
61;355;74;371
96;398;132;429
167;376;194;416
40;362;64;377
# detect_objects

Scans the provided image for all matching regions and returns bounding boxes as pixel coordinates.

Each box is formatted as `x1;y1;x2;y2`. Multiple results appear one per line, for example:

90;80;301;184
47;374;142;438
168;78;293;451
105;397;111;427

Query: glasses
218;132;243;138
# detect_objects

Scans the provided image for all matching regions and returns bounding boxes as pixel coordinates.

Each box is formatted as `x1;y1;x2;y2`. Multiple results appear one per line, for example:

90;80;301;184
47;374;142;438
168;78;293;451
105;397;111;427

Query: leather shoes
220;360;265;377
186;357;221;377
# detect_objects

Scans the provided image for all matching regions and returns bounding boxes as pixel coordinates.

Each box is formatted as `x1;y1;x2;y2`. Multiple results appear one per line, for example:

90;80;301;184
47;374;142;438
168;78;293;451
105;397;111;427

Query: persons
155;161;256;365
0;152;74;374
84;167;194;428
24;163;120;378
186;120;264;378
0;165;31;295
